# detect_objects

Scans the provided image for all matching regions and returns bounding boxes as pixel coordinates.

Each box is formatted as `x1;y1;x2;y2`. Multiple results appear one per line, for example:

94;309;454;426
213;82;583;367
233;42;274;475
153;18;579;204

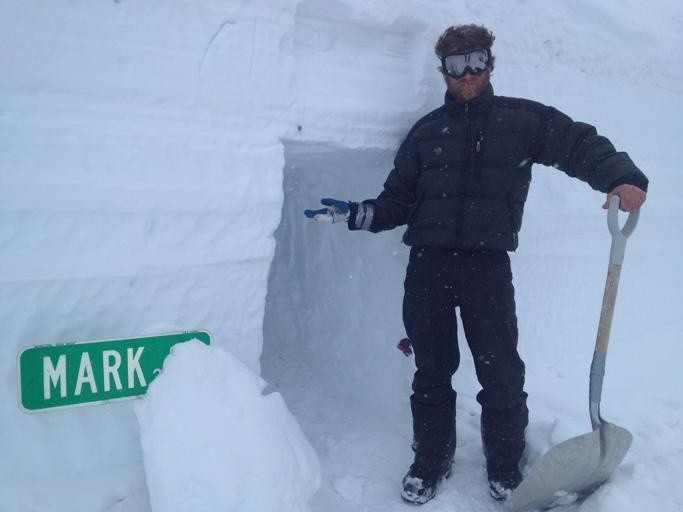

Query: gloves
303;198;351;224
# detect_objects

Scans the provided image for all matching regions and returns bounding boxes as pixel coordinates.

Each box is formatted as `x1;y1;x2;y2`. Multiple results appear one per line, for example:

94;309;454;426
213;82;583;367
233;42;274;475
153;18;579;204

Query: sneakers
486;457;524;501
400;451;454;504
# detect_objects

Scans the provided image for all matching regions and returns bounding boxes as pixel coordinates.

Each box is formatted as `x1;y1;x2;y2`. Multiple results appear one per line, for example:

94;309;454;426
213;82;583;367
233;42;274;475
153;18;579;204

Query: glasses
442;48;491;80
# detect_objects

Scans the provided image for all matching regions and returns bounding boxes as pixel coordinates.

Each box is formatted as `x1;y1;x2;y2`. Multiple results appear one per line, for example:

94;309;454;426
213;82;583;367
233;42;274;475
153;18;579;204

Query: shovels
501;194;640;512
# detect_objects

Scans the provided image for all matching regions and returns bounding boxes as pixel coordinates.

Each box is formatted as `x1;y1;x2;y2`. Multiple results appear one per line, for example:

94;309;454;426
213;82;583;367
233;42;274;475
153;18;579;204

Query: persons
303;25;650;503
